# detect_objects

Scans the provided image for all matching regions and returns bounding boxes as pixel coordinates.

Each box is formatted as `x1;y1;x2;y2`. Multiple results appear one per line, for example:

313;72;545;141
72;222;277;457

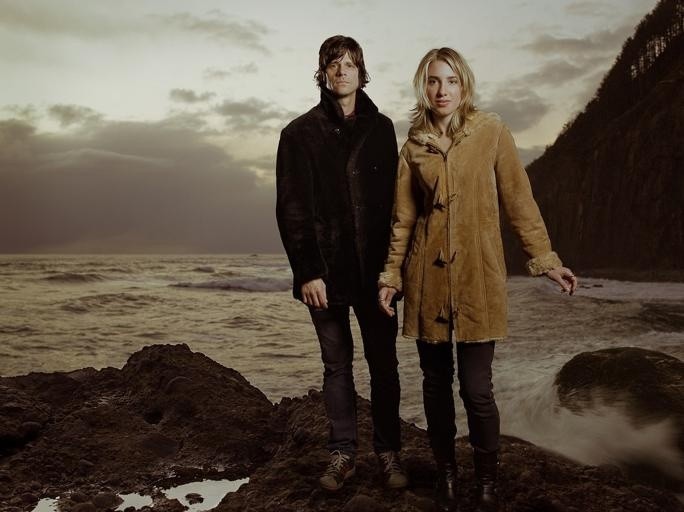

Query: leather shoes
473;479;500;511
434;477;457;512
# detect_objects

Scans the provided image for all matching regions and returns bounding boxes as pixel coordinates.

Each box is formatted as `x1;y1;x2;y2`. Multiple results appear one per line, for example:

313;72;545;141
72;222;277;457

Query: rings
568;274;574;277
377;299;384;301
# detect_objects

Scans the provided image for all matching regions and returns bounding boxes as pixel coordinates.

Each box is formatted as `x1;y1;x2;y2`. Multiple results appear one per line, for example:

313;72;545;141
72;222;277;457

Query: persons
275;35;408;491
378;48;579;512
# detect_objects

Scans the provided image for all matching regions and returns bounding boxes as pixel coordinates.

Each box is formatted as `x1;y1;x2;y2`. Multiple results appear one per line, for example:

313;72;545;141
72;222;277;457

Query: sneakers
378;449;409;488
319;450;356;491
